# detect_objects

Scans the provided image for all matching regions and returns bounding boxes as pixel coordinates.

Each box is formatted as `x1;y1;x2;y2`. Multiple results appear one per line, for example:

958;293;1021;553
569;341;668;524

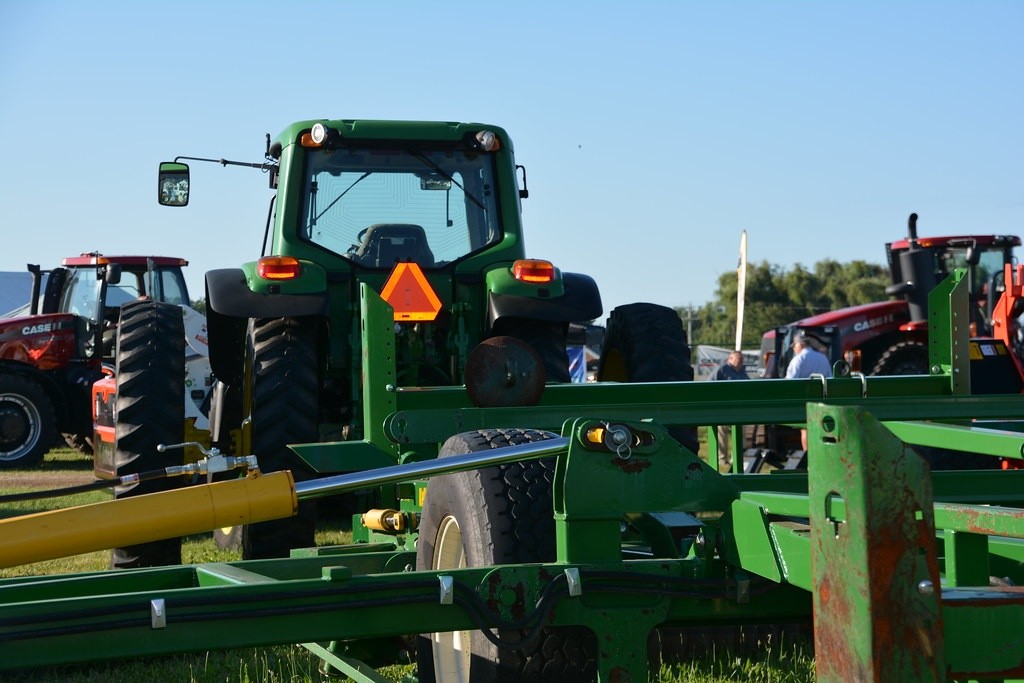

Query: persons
786;333;832;451
708;351;750;465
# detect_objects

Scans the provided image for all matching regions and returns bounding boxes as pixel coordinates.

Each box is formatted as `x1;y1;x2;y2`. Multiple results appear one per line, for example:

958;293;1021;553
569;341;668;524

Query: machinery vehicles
0;247;219;488
0;114;1024;683
742;209;1024;473
